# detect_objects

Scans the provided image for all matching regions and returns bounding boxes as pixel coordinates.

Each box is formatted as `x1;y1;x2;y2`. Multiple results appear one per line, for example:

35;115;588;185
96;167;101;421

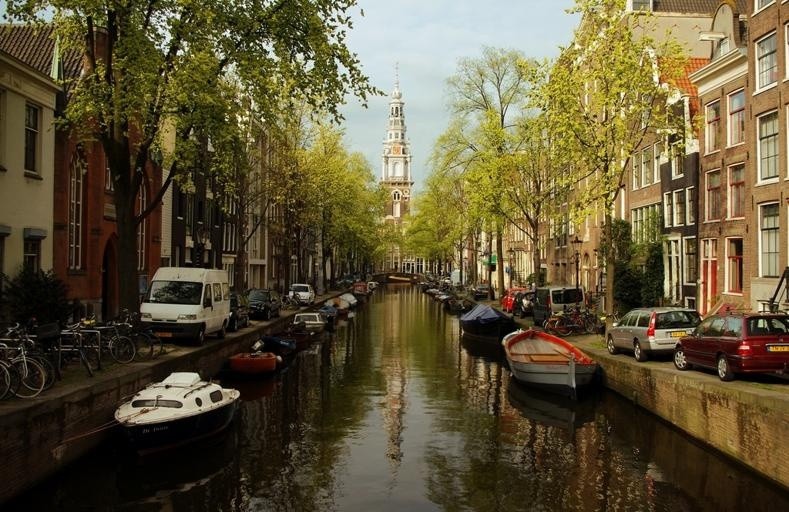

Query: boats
504;372;591;441
226;271;382;380
419;280;598;402
113;370;244;451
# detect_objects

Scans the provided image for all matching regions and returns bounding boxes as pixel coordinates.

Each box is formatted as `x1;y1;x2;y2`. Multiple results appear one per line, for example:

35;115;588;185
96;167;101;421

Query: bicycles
545;306;602;338
0;311;165;403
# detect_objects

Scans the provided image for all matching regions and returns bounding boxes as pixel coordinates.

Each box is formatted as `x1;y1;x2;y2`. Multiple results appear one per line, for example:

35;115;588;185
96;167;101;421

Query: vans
140;265;233;347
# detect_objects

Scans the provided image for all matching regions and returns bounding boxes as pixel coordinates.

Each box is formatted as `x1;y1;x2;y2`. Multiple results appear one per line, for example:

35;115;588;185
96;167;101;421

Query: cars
672;311;789;385
605;305;702;362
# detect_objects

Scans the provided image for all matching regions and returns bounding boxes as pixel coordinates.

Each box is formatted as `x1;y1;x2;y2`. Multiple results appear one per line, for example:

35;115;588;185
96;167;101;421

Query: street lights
478;253;486;282
462;257;468;283
290;254;298;282
506;246;515;288
571;234;581;312
